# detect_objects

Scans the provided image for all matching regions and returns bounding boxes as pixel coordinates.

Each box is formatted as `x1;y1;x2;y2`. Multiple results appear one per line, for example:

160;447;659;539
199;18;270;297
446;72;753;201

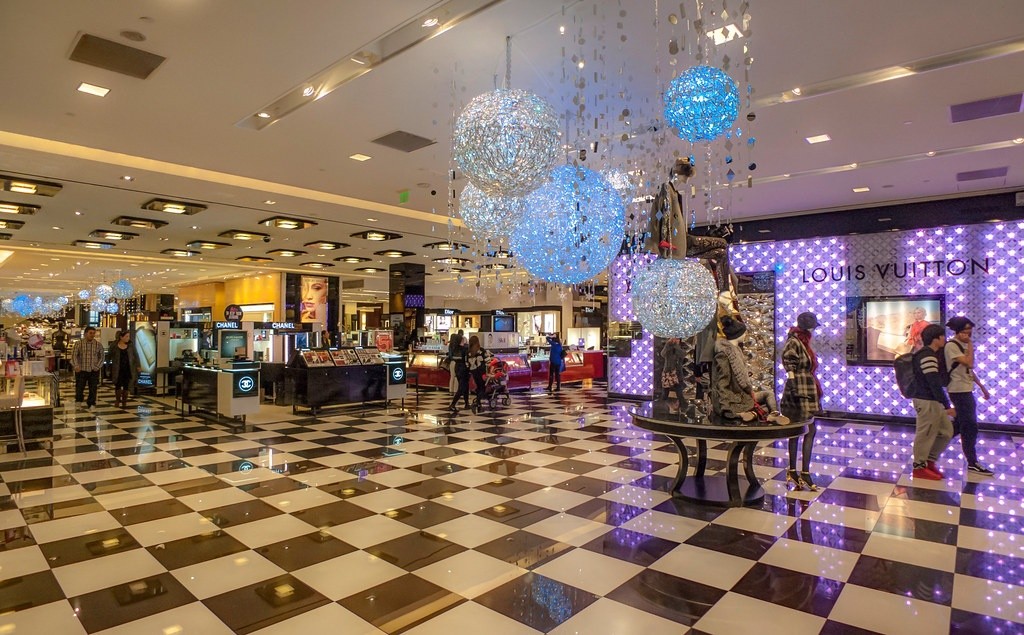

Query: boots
115;389;122;406
554;375;560;391
543;375;553;391
122;391;129;410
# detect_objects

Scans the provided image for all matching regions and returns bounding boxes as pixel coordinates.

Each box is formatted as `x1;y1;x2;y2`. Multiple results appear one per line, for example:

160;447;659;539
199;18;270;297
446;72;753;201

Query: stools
0;374;31;459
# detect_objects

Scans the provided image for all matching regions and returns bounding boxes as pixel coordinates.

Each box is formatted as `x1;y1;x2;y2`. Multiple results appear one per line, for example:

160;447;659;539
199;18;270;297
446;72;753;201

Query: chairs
390;356;418;411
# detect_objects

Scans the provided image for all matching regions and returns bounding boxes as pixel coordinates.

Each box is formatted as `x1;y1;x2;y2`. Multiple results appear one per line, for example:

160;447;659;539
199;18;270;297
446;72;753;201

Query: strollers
473;357;512;410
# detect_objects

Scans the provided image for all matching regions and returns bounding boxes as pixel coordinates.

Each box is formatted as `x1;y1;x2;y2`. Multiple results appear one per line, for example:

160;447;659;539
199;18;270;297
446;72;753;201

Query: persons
711;315;790;425
913;324;956;480
300;275;327;323
321;330;330;347
645;157;738;316
543;336;562;390
51;321;69;353
449;334;490;414
944;317;994;476
783;312;821;491
72;326;105;411
659;338;686;399
106;331;141;408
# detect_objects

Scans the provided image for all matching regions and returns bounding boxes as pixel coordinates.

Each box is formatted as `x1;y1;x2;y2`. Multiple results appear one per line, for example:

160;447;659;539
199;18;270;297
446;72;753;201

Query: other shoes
448;404;460;412
471;402;477;415
465;405;472;409
90;406;97;413
479;407;485;413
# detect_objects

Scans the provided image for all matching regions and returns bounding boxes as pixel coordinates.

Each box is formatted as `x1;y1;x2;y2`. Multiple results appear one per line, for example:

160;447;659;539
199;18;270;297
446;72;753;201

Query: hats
720;315;746;340
672;157;696;177
797;312;821;329
946;317;971;333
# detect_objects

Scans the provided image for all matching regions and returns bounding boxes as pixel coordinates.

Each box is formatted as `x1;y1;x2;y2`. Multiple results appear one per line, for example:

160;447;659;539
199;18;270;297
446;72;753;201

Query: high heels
786;469;807;488
735;411;756;422
719;291;738;314
767;411;790;425
799;470;818;491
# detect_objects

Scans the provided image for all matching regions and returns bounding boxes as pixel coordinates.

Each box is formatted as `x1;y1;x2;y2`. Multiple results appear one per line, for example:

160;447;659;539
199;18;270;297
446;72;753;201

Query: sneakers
76;402;82;411
914;467;940;480
926;460;944;477
968;462;994;476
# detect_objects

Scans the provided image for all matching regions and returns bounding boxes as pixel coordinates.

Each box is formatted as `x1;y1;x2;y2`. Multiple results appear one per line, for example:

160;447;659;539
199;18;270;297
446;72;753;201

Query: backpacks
894;347;941;398
936;340;966;386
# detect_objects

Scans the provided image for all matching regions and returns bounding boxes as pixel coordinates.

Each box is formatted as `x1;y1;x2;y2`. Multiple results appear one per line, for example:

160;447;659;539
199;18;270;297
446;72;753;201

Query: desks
627;396;815;509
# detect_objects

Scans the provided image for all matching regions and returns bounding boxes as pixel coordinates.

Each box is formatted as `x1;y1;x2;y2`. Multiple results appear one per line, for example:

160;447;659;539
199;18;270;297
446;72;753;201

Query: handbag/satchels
561;350;567;359
